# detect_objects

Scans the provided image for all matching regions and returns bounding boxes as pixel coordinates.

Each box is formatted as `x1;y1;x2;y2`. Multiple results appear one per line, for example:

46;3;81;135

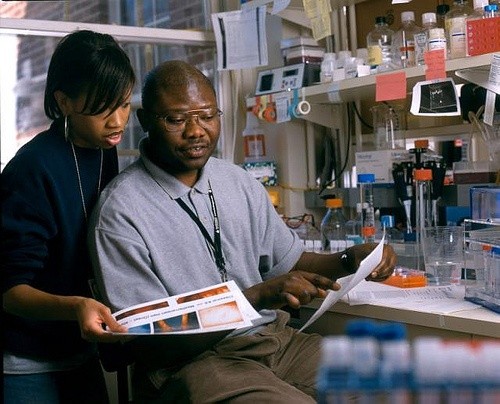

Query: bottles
366;16;395;74
396;0;477;68
241;111;266;164
320;48;369;82
321;319;499;404
482;5;499;18
320;198;406;275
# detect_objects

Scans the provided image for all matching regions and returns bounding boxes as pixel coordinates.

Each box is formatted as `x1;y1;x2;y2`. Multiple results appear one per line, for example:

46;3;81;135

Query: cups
370;105;405;149
420;227;465;287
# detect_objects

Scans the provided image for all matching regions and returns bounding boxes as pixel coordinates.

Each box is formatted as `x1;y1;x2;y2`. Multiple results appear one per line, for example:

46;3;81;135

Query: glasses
145;108;224;132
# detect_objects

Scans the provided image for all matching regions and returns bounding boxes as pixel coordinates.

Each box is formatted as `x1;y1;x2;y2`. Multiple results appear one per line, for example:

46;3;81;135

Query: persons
89;61;397;404
0;31;135;404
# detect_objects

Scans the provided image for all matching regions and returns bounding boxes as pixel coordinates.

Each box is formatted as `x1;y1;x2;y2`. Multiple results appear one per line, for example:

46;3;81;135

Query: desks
301;265;500;338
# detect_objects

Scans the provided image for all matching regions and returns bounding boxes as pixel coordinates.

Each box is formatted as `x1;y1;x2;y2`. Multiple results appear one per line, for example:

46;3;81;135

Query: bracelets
339;247;357;275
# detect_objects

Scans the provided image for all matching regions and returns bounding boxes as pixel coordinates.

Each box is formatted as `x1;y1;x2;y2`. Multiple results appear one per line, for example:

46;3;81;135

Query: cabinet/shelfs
241;0;500;129
